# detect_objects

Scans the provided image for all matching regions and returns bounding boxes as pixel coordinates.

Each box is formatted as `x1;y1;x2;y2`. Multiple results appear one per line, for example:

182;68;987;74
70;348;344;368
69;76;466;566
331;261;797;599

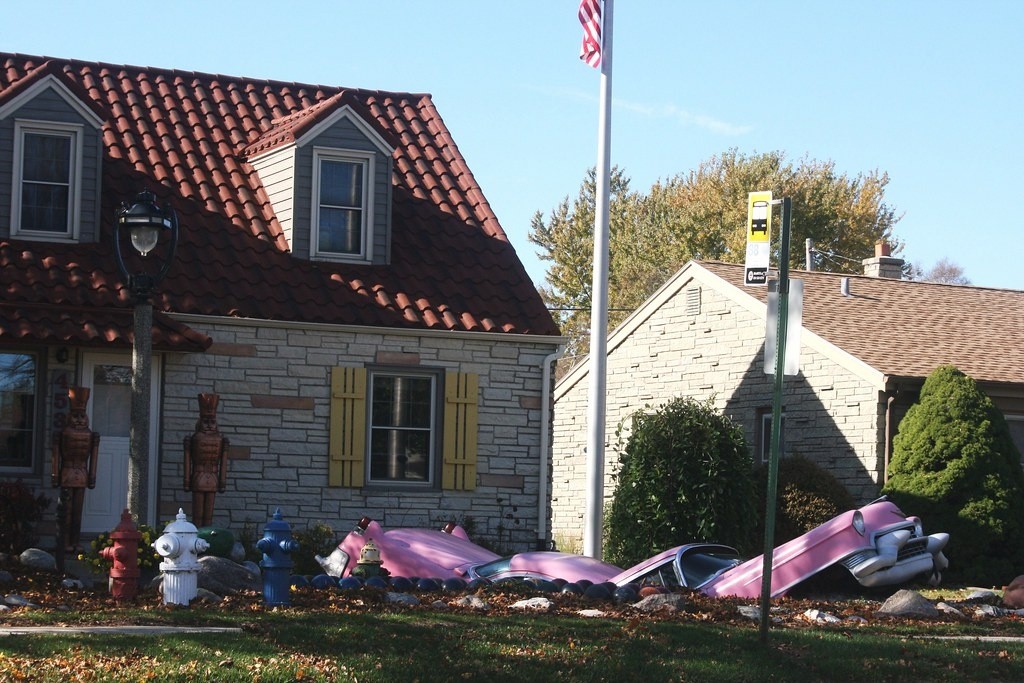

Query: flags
578;0;600;69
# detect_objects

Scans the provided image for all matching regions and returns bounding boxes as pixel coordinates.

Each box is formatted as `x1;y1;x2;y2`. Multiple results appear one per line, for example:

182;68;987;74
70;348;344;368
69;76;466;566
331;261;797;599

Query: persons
183;394;230;527
51;387;100;552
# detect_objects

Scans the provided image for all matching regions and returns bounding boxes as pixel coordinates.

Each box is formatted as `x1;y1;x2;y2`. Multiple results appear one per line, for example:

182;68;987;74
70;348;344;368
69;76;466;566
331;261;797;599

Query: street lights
111;182;179;531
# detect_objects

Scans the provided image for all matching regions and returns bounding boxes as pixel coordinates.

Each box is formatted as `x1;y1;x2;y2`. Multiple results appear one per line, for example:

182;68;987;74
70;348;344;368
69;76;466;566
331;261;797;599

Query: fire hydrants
255;507;300;608
348;537;384;582
150;507;211;607
98;508;144;602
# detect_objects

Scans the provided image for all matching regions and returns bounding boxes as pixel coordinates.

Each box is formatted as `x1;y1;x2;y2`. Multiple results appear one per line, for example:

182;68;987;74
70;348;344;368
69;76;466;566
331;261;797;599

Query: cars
314;494;950;606
